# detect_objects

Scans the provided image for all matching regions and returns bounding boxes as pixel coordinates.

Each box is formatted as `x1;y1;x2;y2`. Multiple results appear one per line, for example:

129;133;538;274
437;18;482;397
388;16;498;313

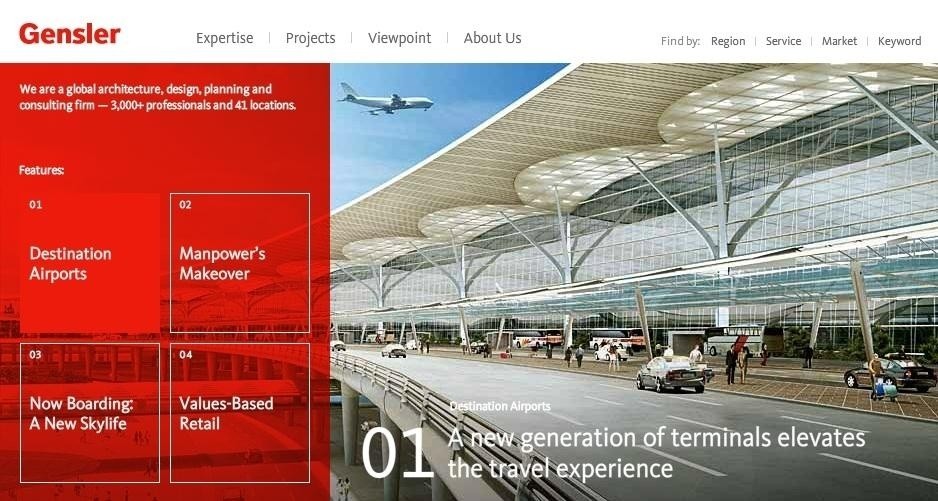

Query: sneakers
870;394;883;401
728;381;735;385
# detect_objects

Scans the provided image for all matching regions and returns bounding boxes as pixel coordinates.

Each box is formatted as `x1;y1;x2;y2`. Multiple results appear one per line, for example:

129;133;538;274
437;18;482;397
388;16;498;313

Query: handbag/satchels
875;382;886;395
882;384;898;395
726;368;730;375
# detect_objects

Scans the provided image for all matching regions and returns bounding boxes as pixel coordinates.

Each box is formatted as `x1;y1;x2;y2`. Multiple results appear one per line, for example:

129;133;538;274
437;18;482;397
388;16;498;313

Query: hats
873;354;880;362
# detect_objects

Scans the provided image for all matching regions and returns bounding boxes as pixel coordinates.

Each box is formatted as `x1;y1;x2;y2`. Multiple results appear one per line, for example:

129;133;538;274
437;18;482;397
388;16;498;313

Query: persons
760;343;769;366
419;338;431;353
899;344;907;360
796;344;813;368
599;339;607;347
565;344;584;368
655;342;674;356
869;353;885;389
547;342;552;358
726;344;749;385
336;477;352;501
484;341;492;358
506;345;512;358
608;345;620;372
690;344;703;362
